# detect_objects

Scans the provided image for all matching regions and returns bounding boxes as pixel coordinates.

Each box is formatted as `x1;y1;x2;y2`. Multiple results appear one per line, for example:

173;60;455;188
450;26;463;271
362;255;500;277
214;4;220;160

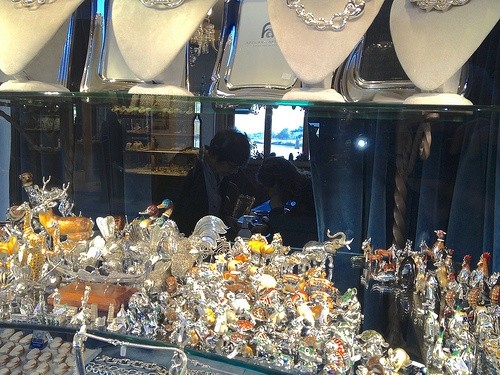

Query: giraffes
16;201;46;252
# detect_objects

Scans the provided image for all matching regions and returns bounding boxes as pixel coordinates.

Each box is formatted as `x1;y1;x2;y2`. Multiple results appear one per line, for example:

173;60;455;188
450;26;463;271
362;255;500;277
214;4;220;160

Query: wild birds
138;199;174;240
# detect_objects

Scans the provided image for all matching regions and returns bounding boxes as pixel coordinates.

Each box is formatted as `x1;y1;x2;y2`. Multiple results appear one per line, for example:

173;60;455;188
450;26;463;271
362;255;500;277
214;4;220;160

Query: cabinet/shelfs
9;99;202;234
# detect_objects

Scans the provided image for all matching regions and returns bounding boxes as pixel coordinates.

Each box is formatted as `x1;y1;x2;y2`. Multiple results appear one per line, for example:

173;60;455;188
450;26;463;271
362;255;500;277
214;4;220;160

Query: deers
37;176;95;243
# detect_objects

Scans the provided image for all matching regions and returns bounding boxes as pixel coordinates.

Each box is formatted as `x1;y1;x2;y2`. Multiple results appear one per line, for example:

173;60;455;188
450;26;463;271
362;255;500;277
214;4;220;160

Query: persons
168;129;250;241
93;112;126;197
133;121;142;131
252;157;318;249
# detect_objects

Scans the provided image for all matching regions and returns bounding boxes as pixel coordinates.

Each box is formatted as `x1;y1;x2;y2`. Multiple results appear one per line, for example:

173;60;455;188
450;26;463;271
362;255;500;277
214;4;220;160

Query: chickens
477;252;500;290
456;253;472;284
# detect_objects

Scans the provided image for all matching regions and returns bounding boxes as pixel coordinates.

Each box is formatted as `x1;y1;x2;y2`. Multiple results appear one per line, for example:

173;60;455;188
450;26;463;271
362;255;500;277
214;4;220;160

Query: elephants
302;229;354;269
